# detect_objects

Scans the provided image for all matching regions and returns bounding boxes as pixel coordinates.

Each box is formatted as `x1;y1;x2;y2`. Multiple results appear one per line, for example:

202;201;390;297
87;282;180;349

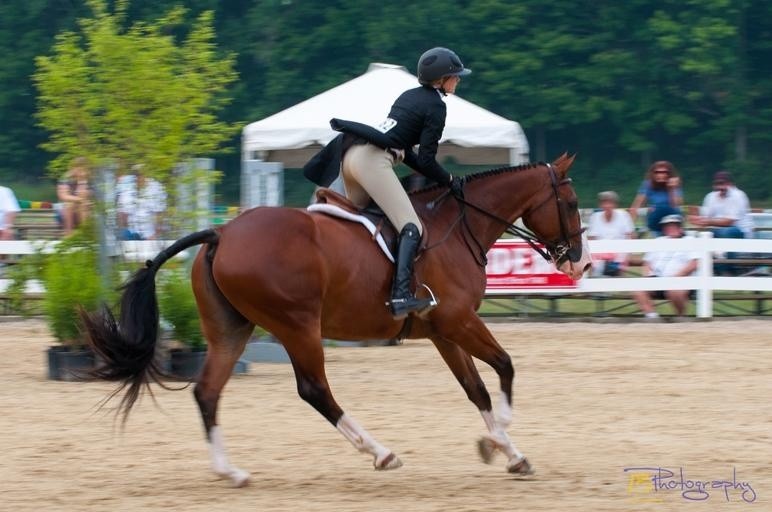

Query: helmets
418;47;472;83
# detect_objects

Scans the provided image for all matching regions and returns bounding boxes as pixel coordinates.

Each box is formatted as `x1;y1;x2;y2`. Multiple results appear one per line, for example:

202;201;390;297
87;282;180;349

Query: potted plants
44;250;210;382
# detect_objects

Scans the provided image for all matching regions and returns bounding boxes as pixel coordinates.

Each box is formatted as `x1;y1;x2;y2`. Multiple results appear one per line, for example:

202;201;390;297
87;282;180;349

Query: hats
659;214;683;223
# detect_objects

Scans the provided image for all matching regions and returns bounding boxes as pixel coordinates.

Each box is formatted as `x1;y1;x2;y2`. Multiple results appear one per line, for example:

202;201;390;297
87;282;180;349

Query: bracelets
449;172;453;182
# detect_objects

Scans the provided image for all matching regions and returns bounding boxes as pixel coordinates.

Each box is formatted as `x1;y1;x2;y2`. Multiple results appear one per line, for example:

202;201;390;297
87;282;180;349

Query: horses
77;149;595;489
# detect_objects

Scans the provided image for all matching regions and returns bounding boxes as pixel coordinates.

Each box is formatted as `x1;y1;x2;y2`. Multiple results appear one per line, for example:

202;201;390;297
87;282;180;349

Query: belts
369;142;397;160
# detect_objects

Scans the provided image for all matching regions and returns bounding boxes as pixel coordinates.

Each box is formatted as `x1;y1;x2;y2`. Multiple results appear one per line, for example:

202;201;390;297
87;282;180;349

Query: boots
391;223;439;321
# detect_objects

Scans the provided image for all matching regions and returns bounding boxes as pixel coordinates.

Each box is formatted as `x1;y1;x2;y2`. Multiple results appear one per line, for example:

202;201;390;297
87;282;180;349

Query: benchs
0;192;772;321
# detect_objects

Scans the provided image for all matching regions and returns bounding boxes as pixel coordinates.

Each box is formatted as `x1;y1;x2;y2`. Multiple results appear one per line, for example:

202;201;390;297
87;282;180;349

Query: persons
302;48;472;321
0;186;22;279
586;161;755;319
57;154;168;240
